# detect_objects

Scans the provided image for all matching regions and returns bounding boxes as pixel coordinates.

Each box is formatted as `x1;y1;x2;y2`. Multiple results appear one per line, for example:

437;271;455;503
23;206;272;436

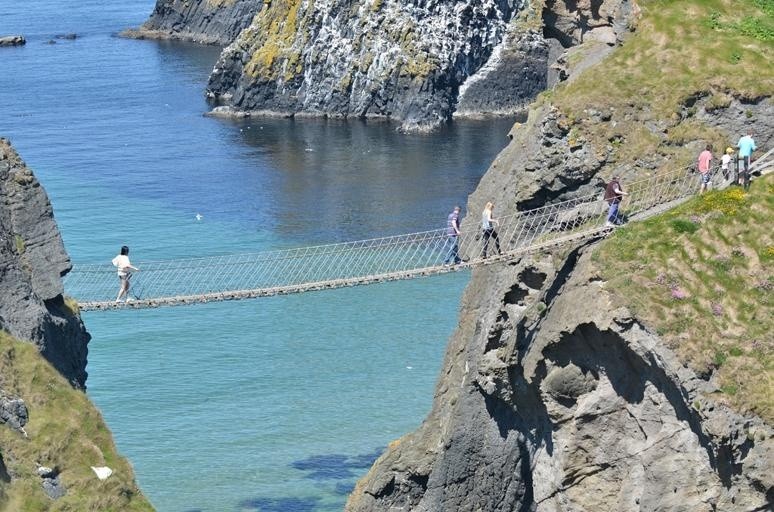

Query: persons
441;206;466;267
603;175;629;227
697;144;713;195
736;129;758;183
111;245;139;304
481;201;504;259
720;147;735;184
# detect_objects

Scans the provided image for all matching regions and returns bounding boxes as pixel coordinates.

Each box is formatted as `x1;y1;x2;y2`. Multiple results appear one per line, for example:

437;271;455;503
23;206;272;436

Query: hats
726;148;734;153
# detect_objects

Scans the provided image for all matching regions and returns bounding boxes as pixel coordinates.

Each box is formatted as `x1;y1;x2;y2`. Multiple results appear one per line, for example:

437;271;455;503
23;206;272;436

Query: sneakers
116;299;134;303
